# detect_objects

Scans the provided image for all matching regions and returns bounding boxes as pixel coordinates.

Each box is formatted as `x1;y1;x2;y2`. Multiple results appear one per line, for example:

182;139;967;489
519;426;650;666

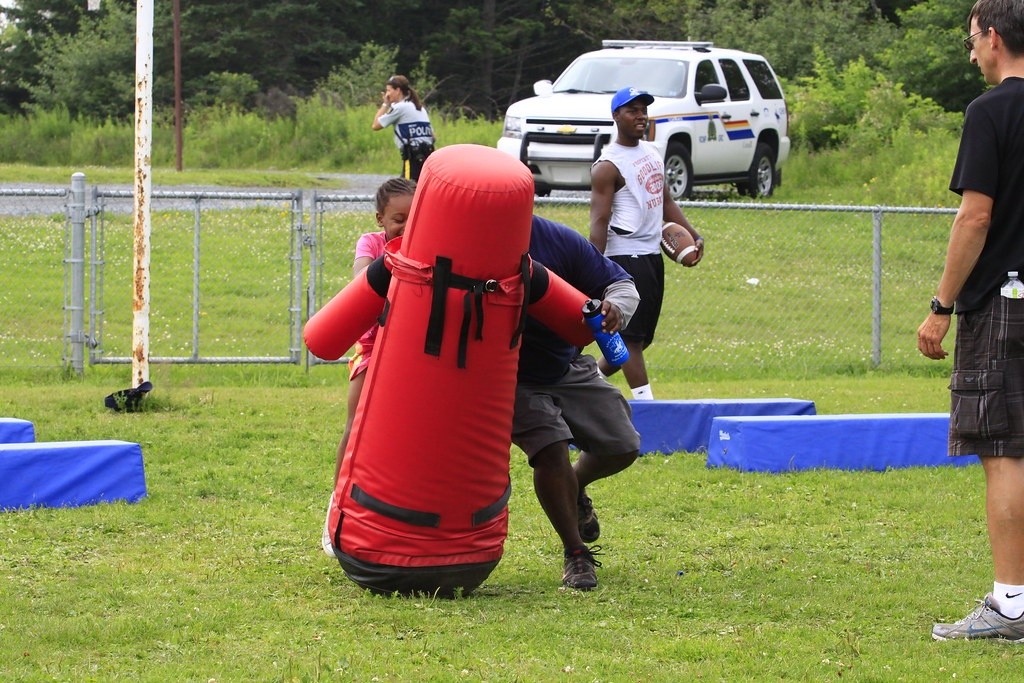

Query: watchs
929;296;955;315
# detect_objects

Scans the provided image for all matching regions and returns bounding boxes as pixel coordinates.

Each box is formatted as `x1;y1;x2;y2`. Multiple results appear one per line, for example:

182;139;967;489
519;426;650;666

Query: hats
611;87;654;119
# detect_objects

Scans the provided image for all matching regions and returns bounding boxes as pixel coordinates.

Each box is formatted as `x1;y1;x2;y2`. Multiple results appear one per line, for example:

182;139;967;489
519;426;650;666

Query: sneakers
932;592;1024;643
562;546;597;590
577;486;601;543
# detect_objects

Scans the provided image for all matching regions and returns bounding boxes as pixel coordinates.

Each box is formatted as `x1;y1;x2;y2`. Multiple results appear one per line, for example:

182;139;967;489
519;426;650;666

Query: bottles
581;298;630;365
1000;270;1024;299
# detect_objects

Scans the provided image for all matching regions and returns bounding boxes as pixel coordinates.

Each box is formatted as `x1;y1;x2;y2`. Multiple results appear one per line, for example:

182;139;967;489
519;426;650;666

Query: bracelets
694;237;705;242
383;103;391;108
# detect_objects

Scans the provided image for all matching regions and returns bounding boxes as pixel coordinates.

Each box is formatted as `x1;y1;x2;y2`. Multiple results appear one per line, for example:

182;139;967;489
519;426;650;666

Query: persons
916;0;1023;647
588;85;707;401
321;178;417;555
512;216;642;593
370;74;437;182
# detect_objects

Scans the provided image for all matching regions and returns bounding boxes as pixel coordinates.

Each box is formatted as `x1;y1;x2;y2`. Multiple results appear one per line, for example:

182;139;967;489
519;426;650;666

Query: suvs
497;39;789;196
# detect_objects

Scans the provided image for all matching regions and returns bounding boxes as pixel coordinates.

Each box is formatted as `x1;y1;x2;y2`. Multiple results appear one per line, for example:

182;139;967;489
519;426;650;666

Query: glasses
389;76;397;90
963;27;989;50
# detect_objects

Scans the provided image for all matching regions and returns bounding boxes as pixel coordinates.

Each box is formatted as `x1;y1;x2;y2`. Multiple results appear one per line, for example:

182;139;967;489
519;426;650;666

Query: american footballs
660;222;697;266
